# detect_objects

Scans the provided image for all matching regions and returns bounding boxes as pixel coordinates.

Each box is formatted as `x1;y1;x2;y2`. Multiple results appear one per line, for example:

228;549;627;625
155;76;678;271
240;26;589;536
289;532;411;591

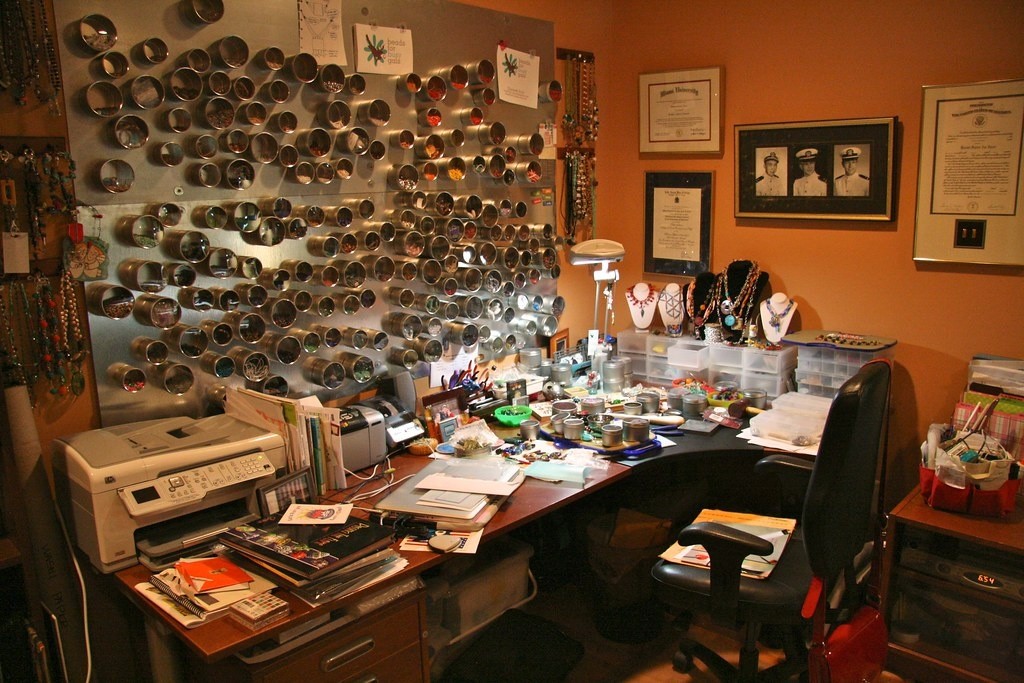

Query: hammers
727;398;765;419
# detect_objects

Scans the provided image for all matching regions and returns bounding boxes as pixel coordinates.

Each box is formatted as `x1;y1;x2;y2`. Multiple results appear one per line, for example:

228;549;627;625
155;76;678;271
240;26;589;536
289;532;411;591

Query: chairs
651;358;892;683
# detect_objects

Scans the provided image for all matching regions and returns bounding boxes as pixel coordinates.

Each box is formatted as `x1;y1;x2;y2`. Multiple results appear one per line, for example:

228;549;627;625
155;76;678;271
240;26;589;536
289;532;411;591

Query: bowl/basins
493;405;533;426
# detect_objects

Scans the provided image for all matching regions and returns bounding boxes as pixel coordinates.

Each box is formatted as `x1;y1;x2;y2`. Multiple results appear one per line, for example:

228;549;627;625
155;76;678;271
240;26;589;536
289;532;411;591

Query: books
135;557;274;630
216;505;396;600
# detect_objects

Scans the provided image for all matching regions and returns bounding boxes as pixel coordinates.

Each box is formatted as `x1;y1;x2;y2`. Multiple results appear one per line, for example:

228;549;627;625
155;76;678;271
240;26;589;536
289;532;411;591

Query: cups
78;0;708;447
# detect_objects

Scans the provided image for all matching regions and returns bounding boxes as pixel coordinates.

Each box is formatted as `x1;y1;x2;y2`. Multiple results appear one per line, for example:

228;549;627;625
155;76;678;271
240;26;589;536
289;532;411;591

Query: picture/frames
732;115;896;222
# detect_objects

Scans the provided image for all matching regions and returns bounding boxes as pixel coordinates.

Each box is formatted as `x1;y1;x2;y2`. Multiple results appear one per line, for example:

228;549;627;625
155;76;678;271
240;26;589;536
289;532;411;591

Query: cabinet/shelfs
891;466;1023;683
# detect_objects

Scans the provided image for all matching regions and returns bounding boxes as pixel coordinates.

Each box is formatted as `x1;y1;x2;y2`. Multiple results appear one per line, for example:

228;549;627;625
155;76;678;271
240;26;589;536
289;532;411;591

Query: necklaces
626;283;655;309
767;296;793;327
0;151;86;411
0;0;63;117
686;258;761;345
559;52;599;244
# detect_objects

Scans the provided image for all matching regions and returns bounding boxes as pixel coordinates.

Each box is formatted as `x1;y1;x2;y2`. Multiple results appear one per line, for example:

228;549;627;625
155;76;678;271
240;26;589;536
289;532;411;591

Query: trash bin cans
586;512;664;645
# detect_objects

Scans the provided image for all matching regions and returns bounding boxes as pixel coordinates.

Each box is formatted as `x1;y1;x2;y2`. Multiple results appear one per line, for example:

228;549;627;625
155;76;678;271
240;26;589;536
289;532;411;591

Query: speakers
375;370;416;415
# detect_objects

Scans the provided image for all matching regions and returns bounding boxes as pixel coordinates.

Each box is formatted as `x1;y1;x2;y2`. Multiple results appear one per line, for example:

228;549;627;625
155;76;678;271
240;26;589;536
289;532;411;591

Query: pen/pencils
551;436;577;446
652;424;684;436
961;399;1000;434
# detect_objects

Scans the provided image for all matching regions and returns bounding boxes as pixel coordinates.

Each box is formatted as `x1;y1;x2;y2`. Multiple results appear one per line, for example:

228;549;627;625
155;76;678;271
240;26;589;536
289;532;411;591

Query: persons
755;149;786;196
834;147;869;196
792;148;828;196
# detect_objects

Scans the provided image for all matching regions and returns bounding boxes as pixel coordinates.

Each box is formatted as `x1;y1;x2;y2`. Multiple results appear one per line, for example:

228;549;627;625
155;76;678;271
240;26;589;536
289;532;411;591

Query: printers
334;404;386;476
53;414;287;575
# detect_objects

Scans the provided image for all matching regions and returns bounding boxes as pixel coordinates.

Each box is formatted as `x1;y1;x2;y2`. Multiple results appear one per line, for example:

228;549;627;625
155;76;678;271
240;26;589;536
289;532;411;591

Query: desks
102;394;850;683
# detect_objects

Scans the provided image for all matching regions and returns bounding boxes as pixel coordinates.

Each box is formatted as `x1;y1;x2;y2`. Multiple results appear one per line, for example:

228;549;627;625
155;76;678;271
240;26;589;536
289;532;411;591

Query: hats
764;151;778;163
795;149;817;159
841;147;861;159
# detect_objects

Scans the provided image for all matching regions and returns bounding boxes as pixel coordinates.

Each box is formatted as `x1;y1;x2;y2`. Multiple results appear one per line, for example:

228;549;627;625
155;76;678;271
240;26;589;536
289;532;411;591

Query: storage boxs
438;546;535;635
619;325;880;415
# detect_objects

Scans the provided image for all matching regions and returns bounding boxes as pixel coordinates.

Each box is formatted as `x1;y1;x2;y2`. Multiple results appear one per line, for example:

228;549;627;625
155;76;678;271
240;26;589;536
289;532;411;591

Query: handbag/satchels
809;605;886;683
